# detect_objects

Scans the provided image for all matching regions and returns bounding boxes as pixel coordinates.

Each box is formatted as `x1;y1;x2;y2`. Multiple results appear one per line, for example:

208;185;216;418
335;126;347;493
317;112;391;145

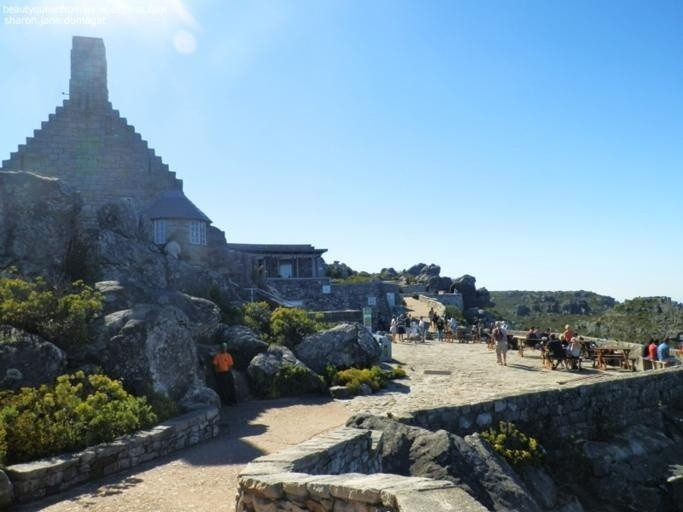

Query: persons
389;307;510;343
641;337;682;365
527;324;581;370
212;342;237;406
377;313;385;330
493;321;508;366
162;235;180;258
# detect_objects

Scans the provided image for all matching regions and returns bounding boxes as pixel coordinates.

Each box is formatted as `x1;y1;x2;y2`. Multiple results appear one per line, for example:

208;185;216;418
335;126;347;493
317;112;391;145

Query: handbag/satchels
495;329;503;341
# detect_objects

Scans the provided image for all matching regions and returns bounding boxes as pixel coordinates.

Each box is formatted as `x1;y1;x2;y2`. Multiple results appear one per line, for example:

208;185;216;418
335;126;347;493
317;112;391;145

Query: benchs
516;330;637;370
639;356;674;370
